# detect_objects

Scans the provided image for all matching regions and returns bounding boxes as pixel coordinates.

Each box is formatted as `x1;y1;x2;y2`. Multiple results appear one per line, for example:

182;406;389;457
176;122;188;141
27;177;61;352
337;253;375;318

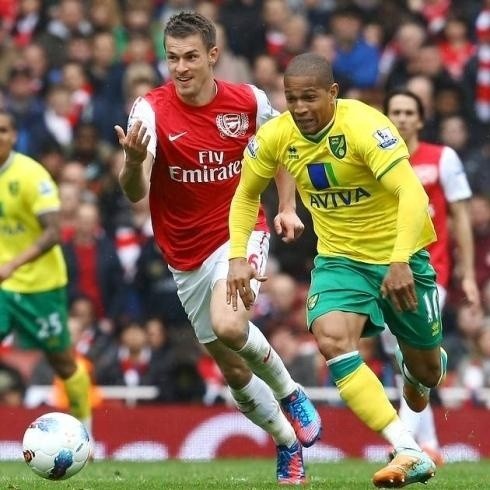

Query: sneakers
373;447;437;488
277;439;308;488
274;382;323;448
394;343;431;412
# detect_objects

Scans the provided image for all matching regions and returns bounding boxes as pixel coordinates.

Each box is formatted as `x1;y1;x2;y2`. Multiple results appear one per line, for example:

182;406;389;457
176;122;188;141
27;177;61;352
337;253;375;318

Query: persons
1;110;95;462
225;52;448;490
382;87;484;470
114;10;325;486
1;1;490;409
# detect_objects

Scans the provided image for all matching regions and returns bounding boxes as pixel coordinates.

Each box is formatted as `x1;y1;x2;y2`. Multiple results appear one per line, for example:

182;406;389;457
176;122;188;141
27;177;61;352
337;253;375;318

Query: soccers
22;412;90;481
